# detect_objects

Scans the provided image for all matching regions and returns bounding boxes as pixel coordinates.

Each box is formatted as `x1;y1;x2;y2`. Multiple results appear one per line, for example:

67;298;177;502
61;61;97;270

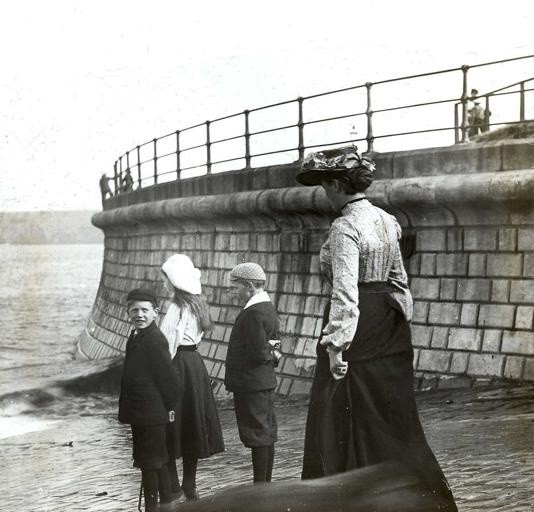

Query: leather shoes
185;487;199;501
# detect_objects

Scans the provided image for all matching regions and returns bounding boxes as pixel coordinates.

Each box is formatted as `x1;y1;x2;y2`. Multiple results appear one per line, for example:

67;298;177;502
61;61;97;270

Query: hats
296;145;377;185
162;255;201;296
126;289;158;309
227;263;267;281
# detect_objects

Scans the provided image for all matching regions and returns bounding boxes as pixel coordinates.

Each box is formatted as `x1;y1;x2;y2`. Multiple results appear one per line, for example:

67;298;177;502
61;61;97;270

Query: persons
472;101;487;132
98;174;115;202
468;88;478;137
121;169;132;192
118;286;183;511
157;255;224;501
297;144;459;511
224;262;282;483
